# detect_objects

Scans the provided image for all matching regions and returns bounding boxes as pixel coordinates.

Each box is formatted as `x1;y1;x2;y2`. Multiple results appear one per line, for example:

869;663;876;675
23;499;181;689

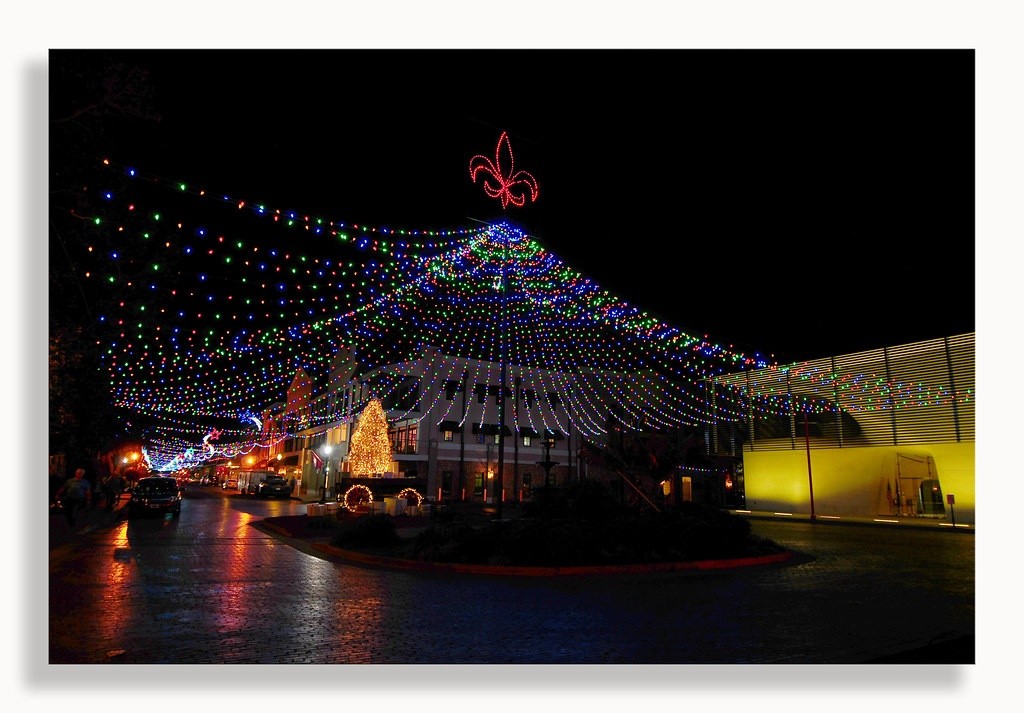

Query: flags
311;450;324;470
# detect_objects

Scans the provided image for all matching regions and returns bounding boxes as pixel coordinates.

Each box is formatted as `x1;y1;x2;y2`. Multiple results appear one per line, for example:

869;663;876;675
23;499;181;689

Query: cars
222;480;237;493
255;475;292;500
130;477;181;516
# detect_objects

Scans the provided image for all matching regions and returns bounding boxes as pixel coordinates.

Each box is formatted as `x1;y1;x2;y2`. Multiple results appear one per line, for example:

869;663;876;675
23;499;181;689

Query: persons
199;475;220;488
289;475;297;494
100;462;122;511
56;468;91;529
